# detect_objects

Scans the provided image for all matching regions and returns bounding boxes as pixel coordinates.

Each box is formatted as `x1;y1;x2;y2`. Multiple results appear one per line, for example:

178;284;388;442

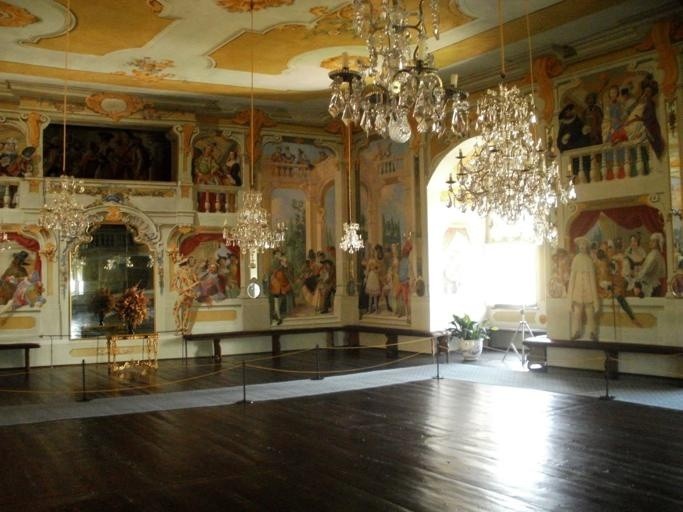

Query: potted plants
115;280;151;335
86;287;116;328
443;310;501;364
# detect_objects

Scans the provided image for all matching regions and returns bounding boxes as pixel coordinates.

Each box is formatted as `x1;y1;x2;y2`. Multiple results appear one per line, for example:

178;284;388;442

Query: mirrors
66;203;165;343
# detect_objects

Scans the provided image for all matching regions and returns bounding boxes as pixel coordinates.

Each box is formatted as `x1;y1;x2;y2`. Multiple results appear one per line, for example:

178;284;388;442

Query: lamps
445;2;578;245
338;124;365;253
440;87;472;138
36;26;103;258
328;69;364;127
219;16;289;299
339;1;447;144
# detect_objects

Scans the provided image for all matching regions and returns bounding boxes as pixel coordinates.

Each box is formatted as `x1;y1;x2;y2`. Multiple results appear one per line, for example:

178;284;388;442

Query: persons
0;138;24;208
362;241;414;318
45;128;171;181
557;74;665;182
270;146;328;171
269;249;336;325
0;250;28;305
188;253;239;302
195;146;242;213
567;232;666;341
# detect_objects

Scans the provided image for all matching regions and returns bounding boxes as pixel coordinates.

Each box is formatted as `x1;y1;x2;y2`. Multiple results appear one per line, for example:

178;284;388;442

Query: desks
105;333;158;375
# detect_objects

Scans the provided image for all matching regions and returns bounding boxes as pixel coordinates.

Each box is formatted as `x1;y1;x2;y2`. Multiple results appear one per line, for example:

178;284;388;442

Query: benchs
0;342;40;370
180;324;430;363
523;335;683;380
